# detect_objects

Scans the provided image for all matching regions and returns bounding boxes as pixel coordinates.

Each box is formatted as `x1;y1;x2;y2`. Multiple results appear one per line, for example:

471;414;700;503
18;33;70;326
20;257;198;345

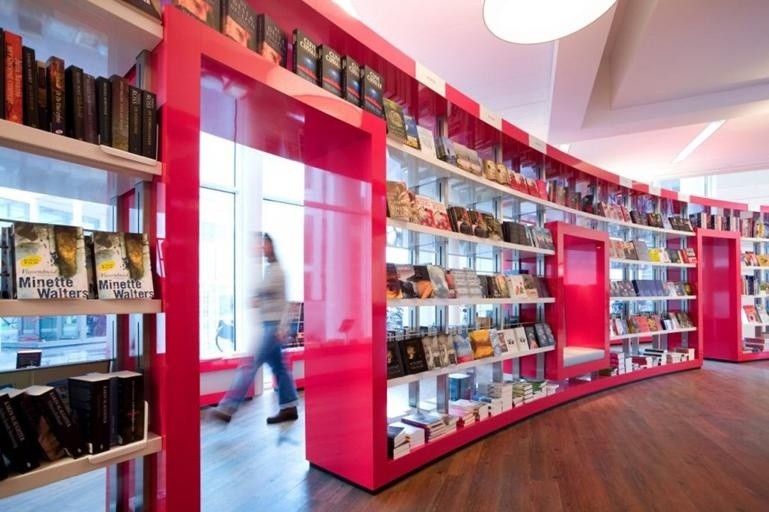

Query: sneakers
266;405;299;424
206;407;232;424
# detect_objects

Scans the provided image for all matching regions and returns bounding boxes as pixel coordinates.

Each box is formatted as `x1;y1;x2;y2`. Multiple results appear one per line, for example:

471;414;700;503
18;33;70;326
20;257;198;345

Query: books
609;240;697;264
1;26;157;158
387;373;559;459
178;1;437;157
741;251;768;353
0;370;144;480
2;222;154;299
599;347;694;376
386;263;552;299
436;136;691;232
387;181;554;251
387;317;555;379
689;212;768;238
609;279;693;296
610;312;693;337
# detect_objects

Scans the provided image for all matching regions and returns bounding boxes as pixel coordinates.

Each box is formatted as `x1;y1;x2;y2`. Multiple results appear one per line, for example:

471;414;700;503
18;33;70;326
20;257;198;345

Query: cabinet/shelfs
0;0;201;510
303;136;560;492
606;205;769;377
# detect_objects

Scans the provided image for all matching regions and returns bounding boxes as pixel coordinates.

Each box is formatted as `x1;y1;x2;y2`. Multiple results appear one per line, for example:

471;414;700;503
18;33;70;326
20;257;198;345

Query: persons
211;233;300;423
